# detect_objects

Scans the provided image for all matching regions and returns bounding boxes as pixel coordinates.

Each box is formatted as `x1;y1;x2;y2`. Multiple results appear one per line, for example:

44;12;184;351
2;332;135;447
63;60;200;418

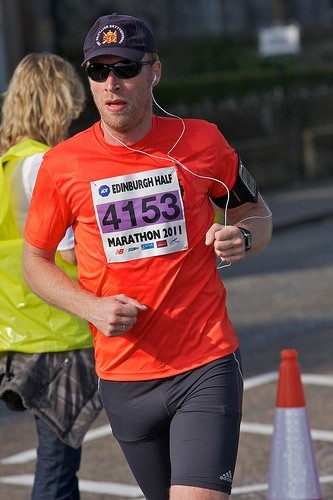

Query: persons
0;54;101;500
22;14;271;500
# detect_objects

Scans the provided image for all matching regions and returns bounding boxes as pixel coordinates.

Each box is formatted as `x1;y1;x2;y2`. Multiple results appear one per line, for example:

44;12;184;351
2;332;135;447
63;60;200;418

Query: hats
81;13;159;62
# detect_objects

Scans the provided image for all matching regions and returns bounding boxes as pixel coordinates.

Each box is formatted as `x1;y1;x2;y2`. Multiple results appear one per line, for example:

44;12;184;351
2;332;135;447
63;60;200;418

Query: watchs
238;227;253;252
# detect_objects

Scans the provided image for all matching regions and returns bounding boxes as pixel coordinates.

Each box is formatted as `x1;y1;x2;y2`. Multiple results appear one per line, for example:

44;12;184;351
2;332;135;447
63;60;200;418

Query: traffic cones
268;349;323;500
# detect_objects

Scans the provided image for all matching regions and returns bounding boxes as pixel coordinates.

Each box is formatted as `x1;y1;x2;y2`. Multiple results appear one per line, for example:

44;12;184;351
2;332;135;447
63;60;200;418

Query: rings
122;324;125;332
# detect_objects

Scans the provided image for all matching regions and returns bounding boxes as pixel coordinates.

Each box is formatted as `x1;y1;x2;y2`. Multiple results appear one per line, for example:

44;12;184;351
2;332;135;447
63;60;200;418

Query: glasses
80;60;154;82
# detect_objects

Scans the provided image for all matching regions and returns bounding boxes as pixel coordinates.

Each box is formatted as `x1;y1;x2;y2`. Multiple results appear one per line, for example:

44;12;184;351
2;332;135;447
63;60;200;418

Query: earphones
151;74;157;84
